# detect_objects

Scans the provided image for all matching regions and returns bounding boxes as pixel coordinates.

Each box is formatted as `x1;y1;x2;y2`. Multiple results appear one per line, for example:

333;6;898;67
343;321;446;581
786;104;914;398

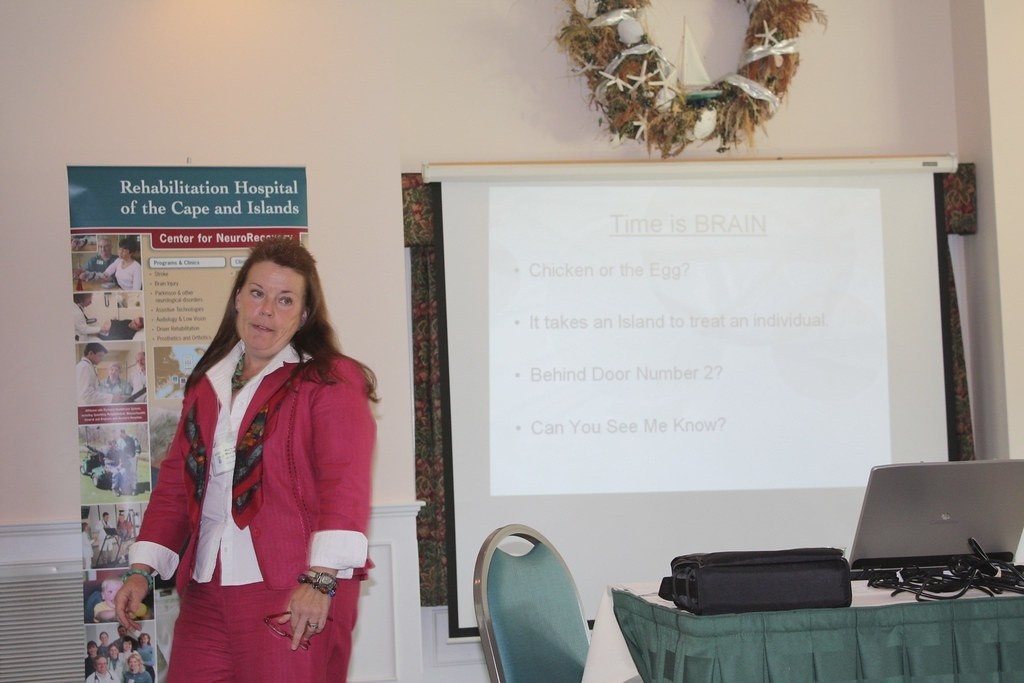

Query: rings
307;621;318;628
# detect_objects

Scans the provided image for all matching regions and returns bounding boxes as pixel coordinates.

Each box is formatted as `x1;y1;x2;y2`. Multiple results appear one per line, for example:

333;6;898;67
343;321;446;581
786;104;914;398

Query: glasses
264;611;333;650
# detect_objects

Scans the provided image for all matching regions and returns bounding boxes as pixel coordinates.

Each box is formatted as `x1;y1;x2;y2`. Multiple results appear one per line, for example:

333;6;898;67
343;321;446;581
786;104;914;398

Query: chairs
472;520;590;683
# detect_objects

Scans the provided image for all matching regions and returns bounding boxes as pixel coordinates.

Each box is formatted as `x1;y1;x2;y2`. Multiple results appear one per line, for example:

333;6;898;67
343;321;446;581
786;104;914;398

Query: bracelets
298;575;336;599
121;569;153;590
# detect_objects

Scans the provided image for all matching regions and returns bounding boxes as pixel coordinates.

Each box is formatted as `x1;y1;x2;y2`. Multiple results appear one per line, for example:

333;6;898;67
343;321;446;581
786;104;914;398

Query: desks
582;571;1024;683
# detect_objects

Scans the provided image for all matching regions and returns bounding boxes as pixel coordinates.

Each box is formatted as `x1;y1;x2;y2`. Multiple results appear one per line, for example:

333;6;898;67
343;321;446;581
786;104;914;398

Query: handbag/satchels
658;548;852;616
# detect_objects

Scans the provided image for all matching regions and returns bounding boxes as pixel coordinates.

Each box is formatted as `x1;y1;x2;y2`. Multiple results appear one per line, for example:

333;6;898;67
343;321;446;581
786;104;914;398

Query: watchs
303;569;337;589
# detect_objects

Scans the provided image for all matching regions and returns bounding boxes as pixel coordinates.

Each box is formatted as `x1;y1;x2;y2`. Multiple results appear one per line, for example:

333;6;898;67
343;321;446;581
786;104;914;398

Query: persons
129;351;147;402
114;236;373;683
99;317;144;340
93;576;146;623
73;293;101;341
85;625;155;683
83;239;115;272
105;428;141;497
104;238;142;289
80;523;96;569
102;362;133;395
77;343;123;405
95;512;132;566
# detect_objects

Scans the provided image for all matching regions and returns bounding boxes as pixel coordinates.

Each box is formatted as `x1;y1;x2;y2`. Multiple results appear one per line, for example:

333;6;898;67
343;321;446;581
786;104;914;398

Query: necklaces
232;357;248;390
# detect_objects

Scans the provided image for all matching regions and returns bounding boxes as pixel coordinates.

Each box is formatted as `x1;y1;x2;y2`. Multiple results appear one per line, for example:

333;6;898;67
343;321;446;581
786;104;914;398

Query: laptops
848;460;1024;581
